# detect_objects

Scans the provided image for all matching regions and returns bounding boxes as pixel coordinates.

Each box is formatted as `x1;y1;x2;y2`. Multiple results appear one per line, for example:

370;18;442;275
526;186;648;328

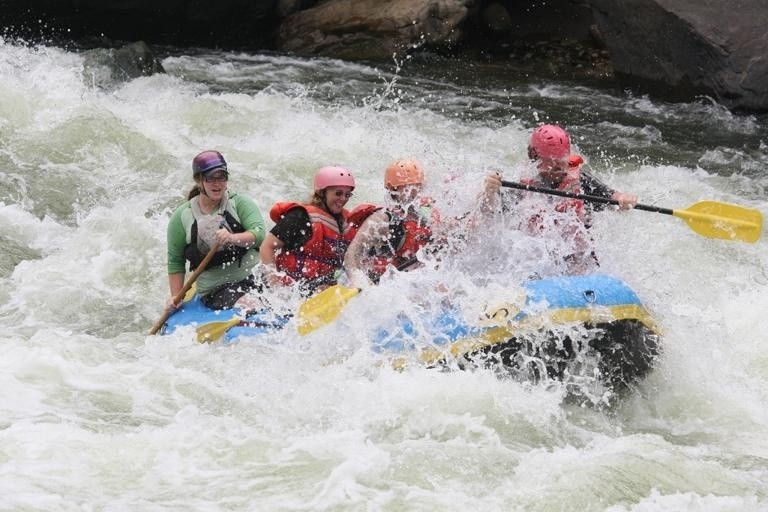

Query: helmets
193;150;226;175
385;161;425;188
532;125;570;159
316;166;356;191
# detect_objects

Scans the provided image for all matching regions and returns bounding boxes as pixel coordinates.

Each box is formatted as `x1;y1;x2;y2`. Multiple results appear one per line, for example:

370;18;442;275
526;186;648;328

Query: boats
163;273;660;407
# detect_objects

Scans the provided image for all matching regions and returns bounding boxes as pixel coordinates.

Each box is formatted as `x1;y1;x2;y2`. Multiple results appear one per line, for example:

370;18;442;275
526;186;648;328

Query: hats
202;166;231;176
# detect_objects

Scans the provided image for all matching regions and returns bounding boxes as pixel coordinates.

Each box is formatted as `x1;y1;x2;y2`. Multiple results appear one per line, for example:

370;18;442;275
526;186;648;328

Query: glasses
206;176;228;182
333;189;353;198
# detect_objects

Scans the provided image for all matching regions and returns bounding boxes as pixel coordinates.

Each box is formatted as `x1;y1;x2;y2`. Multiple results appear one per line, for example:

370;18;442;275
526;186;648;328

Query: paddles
197;309;267;343
501;181;762;244
296;243;450;336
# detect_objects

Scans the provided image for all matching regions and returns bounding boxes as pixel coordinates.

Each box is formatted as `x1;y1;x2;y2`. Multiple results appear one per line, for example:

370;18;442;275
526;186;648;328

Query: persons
168;150;271;311
260;167;375;298
485;124;638;275
344;160;459;287
